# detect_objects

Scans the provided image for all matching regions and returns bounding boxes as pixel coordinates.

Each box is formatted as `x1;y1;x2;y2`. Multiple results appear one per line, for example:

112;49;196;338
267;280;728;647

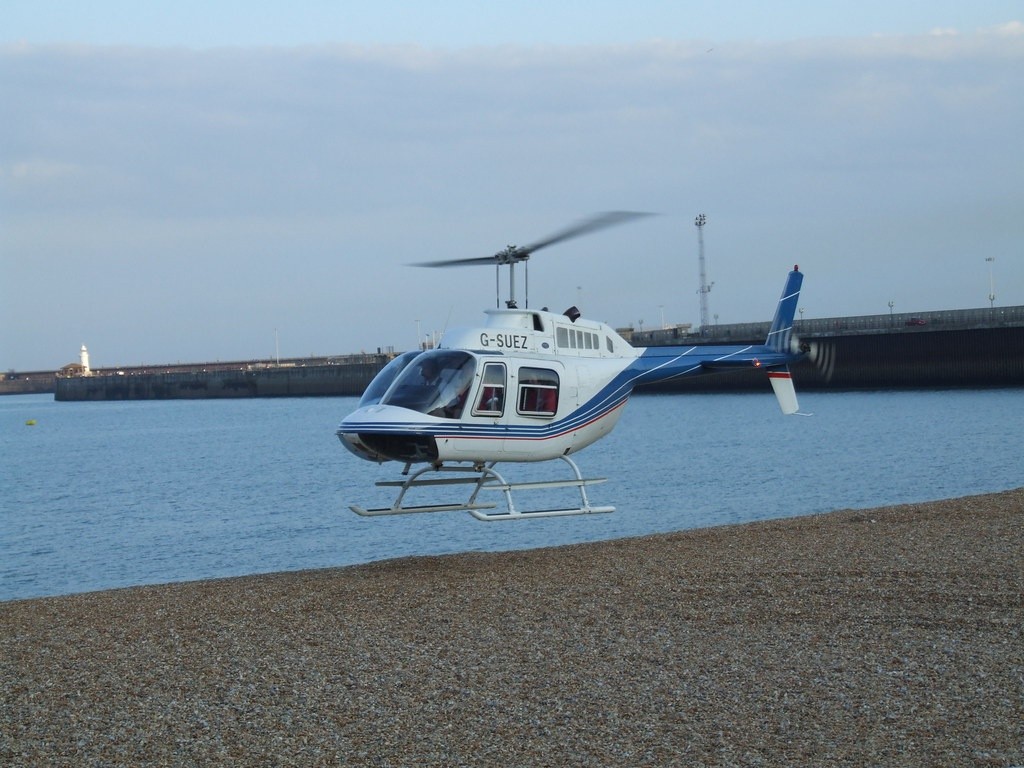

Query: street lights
984;255;996;307
799;308;804;331
888;300;894;327
695;210;705;329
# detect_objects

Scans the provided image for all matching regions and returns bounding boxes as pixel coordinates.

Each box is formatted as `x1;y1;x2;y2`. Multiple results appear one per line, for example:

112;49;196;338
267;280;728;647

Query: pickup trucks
903;317;926;327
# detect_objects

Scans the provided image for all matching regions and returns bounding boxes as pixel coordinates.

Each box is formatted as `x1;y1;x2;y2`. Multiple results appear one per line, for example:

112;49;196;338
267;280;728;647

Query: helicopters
333;211;812;522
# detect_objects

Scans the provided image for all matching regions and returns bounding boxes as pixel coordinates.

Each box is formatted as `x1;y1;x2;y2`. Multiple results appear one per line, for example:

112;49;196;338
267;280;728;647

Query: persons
528;372;557;413
419;358;461;409
458;362;497;410
